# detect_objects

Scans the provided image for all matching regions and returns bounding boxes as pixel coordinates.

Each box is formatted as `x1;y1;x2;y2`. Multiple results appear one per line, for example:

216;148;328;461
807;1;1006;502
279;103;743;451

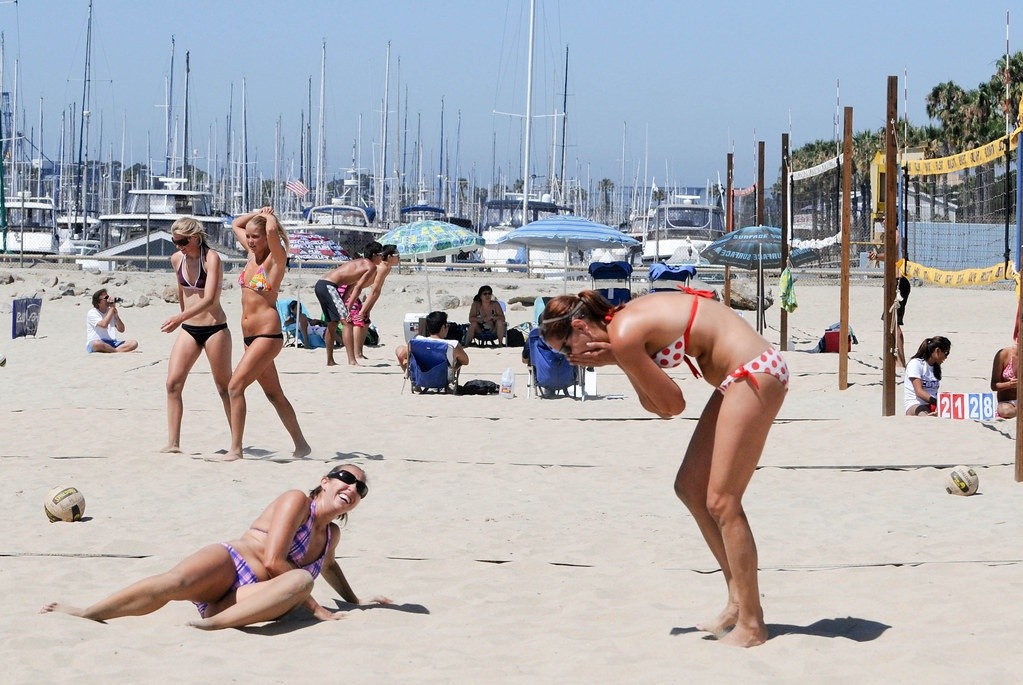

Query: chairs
527;328;590;401
274;298;379;348
471;300;508;348
399;338;462;396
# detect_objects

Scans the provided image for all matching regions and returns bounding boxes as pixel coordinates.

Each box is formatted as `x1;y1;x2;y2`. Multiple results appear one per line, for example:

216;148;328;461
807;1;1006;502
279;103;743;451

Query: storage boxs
403;314;430;346
825;328;851;354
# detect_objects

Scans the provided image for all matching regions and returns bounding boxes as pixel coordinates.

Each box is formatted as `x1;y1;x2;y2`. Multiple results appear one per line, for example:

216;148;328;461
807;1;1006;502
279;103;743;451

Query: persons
336;244;401;361
538;284;790;648
867;213;911;370
284;300;344;349
991;346;1018;418
464;286;506;347
158;217;232;453
395;311;469;392
86;289;138;353
903;336;951;417
522;312;579;396
208;206;312;462
40;464;393;630
314;241;383;366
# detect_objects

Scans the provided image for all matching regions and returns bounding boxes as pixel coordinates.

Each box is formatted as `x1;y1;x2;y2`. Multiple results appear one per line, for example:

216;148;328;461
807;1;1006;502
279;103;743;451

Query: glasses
558;314;586;356
390;253;400;258
443;323;450;328
172;234;191;247
940;349;950;357
98;295;109;300
481;292;492;295
374;252;384;256
326;470;368;499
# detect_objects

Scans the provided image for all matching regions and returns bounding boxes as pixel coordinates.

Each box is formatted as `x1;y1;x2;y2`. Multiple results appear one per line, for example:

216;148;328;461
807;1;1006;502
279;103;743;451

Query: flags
286;174;309;198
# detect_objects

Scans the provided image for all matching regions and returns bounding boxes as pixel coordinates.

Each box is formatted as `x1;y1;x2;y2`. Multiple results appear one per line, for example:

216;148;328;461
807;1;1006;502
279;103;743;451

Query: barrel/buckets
825;330;852;352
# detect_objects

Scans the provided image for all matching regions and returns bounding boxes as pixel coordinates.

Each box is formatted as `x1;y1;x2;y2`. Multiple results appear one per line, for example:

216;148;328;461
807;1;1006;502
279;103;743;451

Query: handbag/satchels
458;379;500;396
506;328;525;346
445;321;472;346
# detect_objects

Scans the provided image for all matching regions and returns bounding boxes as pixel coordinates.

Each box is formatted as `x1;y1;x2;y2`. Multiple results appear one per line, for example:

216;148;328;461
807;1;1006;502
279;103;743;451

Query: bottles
498;368;515;398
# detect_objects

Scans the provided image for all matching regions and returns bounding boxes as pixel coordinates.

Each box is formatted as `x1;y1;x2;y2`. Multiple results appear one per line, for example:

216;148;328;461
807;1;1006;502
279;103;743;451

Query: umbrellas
495;214;640;296
376;220;485;314
700;224;821;334
280;230;352;349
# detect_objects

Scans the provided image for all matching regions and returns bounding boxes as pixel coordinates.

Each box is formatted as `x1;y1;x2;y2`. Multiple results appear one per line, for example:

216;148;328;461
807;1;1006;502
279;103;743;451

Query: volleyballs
944;465;979;496
44;485;86;522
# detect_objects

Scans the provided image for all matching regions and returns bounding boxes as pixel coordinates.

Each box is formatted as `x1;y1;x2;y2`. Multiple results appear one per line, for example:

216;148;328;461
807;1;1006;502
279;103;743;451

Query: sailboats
0;0;741;275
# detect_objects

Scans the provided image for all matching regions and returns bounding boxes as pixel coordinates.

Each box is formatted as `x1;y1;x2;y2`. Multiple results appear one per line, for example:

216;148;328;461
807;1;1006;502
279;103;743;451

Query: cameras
104;295;124;304
